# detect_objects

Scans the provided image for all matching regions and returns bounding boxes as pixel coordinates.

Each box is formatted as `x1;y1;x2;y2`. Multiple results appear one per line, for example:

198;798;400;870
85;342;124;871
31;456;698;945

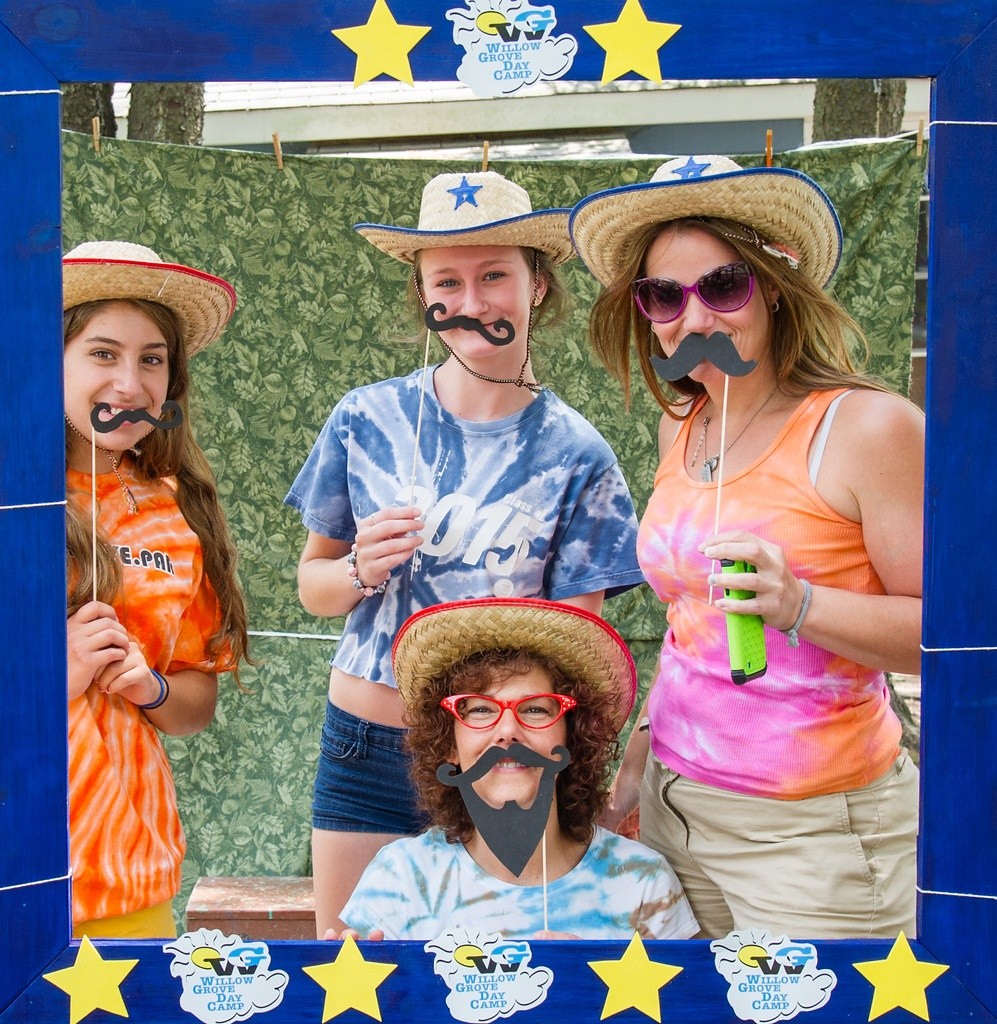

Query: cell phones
719;558;768;685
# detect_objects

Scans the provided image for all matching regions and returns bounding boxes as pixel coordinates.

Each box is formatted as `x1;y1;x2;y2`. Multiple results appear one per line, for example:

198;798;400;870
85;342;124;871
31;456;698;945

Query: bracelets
143;671;170;709
780;579;810;646
348;543;391;598
139;671;165;706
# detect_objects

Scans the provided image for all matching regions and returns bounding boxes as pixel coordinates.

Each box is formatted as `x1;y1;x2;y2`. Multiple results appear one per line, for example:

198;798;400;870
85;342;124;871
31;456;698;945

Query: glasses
440;693;579;729
632;263;755;324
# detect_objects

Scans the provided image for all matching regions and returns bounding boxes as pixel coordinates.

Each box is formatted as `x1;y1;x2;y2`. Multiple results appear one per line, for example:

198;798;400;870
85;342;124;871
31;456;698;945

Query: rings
371;514;378;524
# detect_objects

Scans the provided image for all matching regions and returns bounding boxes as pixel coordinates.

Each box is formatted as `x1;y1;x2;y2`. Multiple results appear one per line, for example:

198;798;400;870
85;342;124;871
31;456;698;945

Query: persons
286;172;646;941
325;599;701;940
569;156;924;940
63;240;255;938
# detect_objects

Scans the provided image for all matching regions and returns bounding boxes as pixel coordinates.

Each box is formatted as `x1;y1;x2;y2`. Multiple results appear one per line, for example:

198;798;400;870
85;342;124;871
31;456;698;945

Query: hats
568;154;842;290
354;172;579;267
390;604;636;738
61;240;235;357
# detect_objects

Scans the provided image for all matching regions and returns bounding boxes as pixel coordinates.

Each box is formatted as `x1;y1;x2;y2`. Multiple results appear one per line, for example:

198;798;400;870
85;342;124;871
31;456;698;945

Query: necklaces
692;387;778;483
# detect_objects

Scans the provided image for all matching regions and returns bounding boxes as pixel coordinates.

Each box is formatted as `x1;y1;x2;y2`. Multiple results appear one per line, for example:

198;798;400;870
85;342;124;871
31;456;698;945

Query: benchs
186;875;315;944
